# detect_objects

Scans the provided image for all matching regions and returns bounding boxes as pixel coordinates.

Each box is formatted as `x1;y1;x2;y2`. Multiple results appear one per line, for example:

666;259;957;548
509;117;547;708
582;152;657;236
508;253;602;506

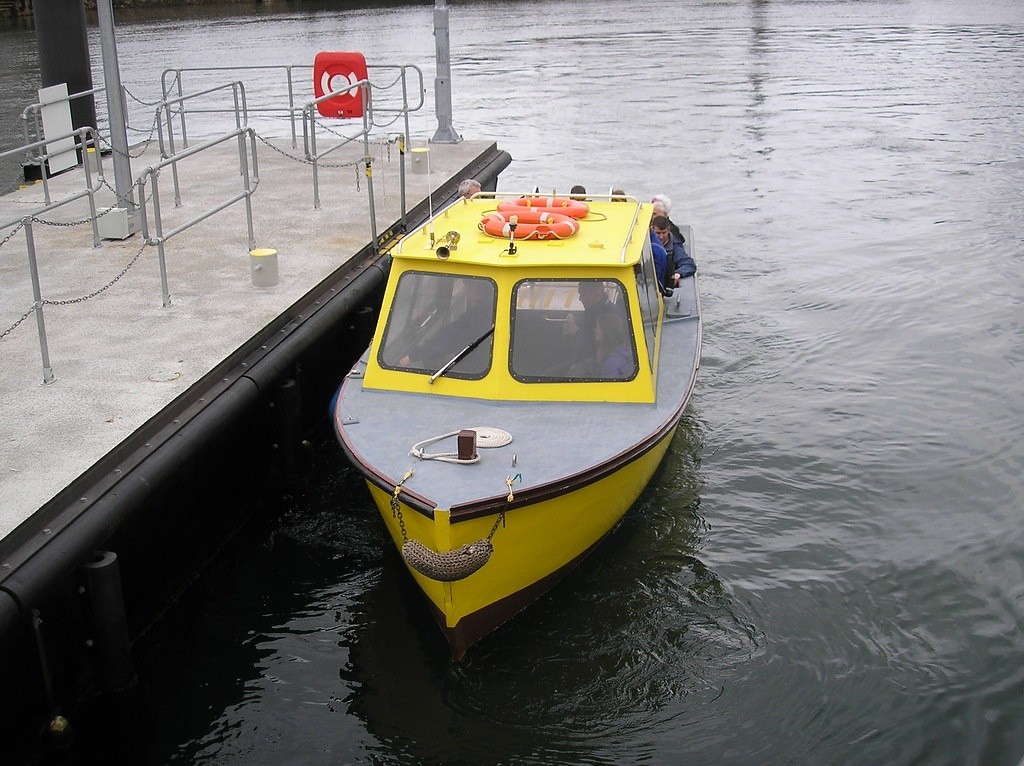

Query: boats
328;140;704;663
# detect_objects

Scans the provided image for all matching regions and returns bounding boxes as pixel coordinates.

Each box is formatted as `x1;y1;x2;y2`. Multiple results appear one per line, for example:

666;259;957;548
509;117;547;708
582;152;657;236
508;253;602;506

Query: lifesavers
482;211;580;239
497;197;589;218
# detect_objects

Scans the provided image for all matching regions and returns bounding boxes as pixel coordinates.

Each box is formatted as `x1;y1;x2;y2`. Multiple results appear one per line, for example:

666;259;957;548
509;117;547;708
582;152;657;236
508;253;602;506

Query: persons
541;281;620;383
640;193;697;294
568;186;590;203
610;190;626;203
398;278;495;373
457;179;481;199
589;315;635;380
